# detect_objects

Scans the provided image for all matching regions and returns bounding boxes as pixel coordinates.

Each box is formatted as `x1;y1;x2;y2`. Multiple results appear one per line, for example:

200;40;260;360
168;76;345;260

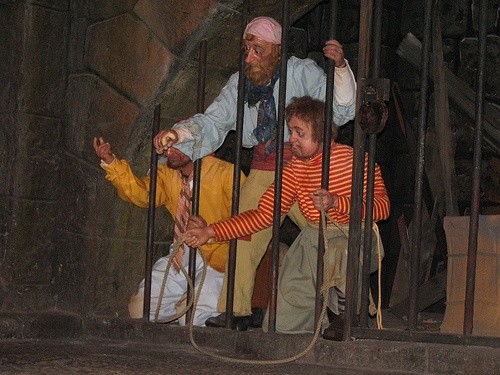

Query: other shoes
205;312;238;330
323;314;345;341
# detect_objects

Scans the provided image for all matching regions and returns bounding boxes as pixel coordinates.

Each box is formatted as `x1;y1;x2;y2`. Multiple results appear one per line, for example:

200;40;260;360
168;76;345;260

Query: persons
153;17;356;327
92;117;246;327
184;96;391;334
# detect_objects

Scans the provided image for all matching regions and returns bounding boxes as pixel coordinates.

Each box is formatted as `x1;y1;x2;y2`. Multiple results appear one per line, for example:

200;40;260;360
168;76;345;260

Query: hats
242;15;282;45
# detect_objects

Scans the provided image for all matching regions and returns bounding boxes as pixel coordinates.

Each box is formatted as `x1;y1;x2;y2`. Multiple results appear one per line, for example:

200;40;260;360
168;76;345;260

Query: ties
171;167;197;274
